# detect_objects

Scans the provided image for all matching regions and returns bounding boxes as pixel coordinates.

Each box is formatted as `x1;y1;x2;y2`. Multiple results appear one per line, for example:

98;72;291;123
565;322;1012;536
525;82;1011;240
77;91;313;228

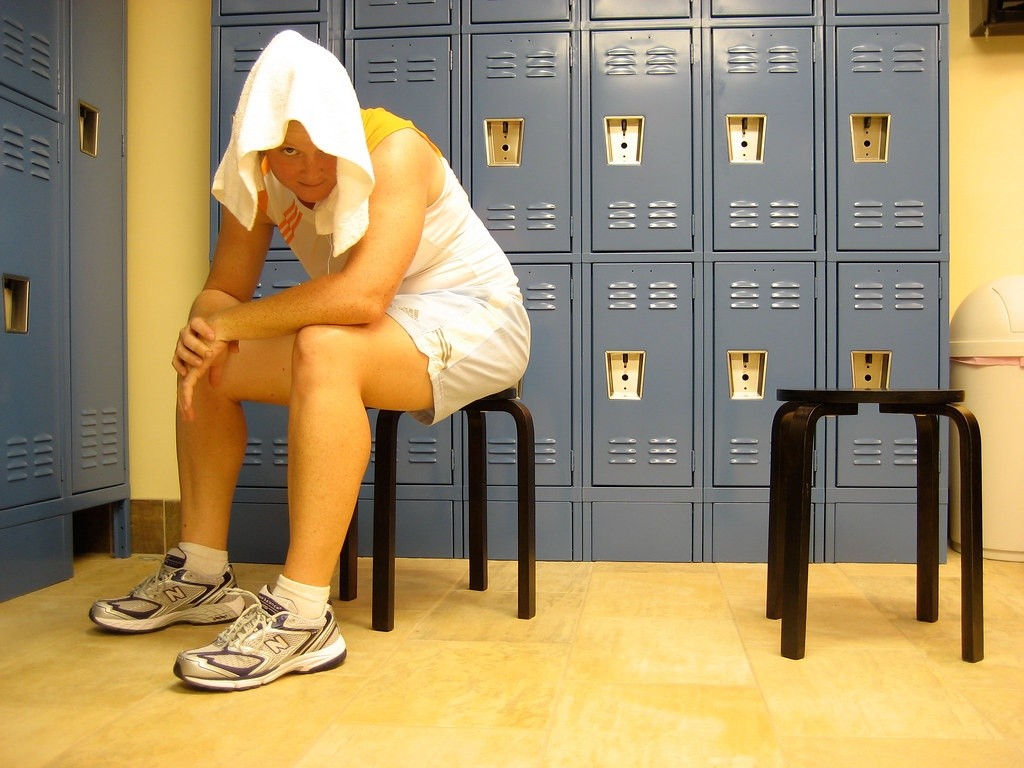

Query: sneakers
89;547;246;632
173;584;347;691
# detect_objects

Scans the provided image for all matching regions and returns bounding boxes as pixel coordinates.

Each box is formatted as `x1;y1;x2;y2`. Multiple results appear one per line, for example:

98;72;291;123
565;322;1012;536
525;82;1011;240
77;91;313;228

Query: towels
211;30;376;258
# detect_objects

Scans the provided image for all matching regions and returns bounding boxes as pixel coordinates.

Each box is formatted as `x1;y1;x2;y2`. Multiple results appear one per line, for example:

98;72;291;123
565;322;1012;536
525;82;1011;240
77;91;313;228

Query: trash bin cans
949;282;1024;565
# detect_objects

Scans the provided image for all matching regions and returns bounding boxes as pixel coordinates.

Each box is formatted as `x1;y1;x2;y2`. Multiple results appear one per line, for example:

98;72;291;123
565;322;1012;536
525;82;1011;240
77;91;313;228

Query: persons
88;29;531;690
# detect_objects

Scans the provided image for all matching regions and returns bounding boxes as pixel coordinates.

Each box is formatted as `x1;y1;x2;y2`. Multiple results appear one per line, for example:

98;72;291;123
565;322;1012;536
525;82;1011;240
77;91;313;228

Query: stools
336;386;538;634
766;388;986;664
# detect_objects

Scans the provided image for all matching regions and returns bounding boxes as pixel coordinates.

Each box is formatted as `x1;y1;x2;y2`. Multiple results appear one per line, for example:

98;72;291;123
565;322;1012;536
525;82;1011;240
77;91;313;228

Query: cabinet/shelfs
209;0;954;562
0;0;131;607
